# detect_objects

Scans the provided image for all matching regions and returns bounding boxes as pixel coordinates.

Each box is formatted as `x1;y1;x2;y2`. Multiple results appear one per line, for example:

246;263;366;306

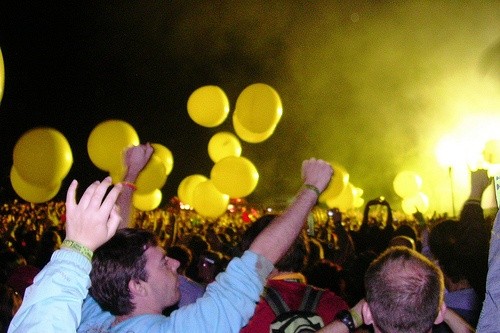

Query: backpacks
261;285;325;333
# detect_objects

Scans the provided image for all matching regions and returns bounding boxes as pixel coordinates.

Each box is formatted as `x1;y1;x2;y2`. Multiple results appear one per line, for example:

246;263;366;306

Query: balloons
10;83;429;218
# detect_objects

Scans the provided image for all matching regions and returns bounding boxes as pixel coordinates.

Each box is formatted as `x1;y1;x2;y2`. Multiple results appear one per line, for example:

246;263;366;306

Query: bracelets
119;179;136;190
350;310;363;327
300;184;320;196
61;238;93;260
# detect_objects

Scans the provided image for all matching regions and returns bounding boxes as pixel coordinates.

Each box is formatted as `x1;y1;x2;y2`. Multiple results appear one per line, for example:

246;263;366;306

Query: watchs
335;310;356;333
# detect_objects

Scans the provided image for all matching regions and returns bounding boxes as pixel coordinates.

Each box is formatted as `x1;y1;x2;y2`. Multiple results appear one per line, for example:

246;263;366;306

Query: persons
0;169;500;333
8;176;122;333
239;214;349;333
76;143;334;333
313;246;476;333
